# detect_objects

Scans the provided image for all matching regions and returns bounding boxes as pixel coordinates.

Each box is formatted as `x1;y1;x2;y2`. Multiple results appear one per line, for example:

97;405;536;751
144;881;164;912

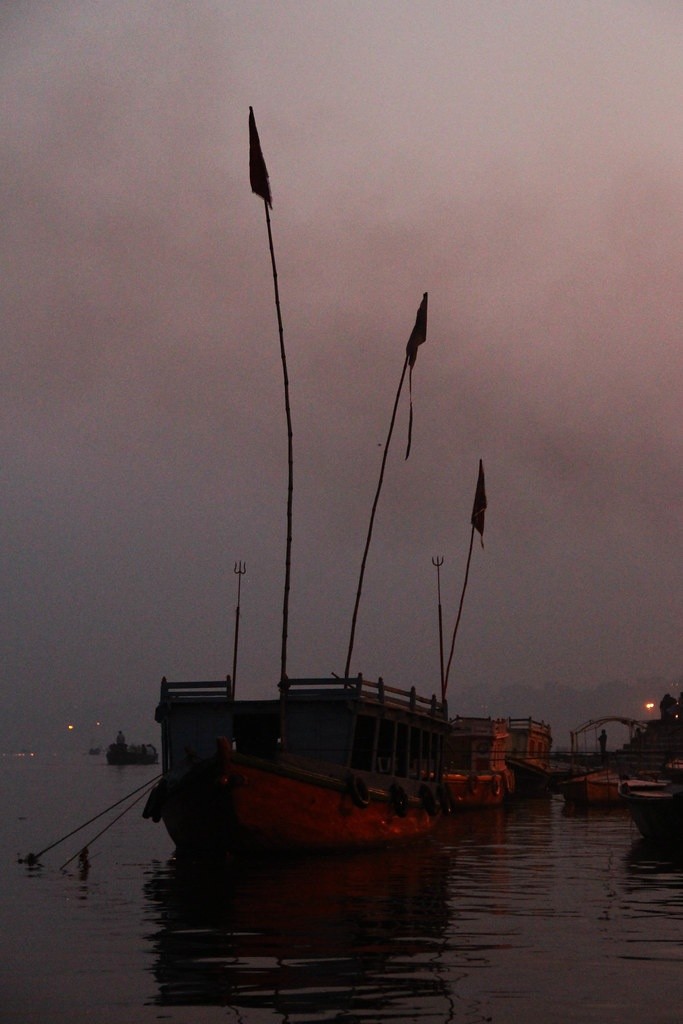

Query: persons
116;730;125;744
597;729;607;752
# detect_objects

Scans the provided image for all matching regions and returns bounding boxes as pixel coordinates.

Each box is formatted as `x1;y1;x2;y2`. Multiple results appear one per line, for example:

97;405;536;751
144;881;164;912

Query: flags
471;459;488;549
248;109;273;210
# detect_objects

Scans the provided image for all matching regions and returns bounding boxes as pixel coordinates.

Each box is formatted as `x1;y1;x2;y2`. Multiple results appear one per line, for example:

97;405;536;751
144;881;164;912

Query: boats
559;715;648;805
617;758;683;849
505;716;562;799
442;714;514;807
141;106;492;863
111;743;158;766
